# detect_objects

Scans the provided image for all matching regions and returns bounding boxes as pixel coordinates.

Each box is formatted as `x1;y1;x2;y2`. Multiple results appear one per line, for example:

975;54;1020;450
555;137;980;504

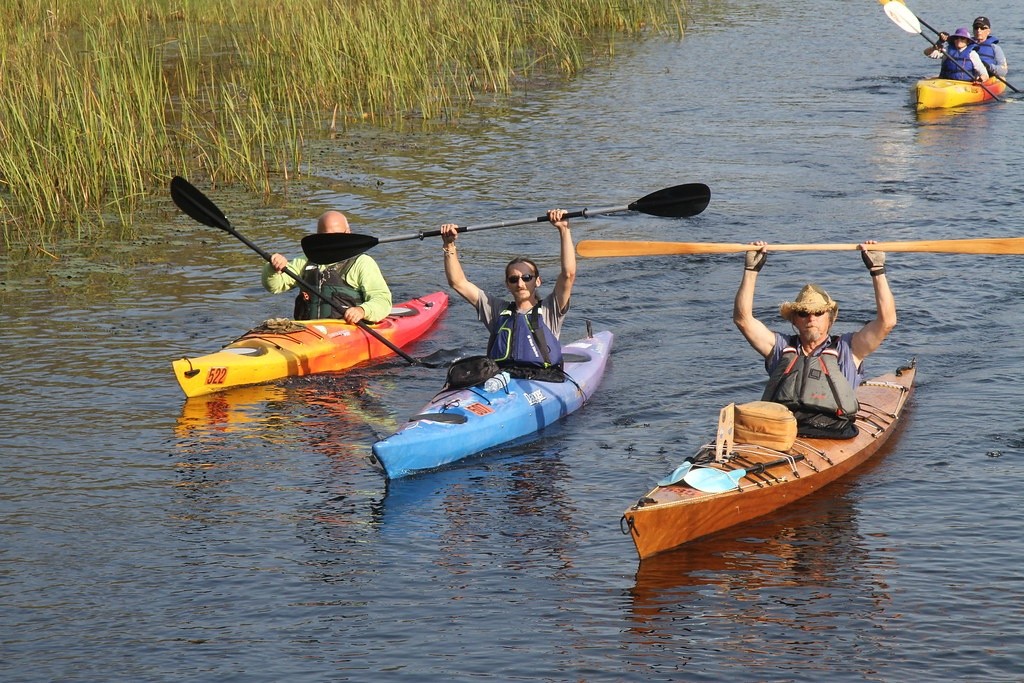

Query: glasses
507;274;537;284
954;36;966;40
793;309;826;317
974;25;990;30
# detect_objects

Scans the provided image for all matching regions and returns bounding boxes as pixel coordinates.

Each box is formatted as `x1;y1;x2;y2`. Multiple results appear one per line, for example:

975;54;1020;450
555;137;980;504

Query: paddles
878;0;1019;103
167;173;419;366
299;181;712;266
573;237;1024;257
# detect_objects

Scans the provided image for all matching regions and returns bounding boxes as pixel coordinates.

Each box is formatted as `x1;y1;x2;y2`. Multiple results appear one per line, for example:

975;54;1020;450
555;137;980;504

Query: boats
915;75;1007;112
372;331;618;480
624;358;916;561
172;292;450;398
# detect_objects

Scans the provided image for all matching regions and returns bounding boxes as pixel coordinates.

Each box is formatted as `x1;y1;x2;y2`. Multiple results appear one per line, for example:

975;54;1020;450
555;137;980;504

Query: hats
971;15;990;28
947;29;978;48
252;317;307;335
778;283;838;324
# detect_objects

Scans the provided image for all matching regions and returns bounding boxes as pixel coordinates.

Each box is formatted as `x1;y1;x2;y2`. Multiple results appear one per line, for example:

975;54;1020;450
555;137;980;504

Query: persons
260;210;392;325
441;208;576;372
924;17;1008;82
733;241;896;438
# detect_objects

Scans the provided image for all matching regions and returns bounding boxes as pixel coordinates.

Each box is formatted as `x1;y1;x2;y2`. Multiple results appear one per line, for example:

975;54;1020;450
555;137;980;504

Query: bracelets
870;267;885;277
937;40;941;44
443;245;455;254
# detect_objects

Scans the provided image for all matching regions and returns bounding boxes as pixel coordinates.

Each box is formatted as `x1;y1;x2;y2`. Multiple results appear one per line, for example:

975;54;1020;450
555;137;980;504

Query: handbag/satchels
734;401;798;451
447;355;500;391
